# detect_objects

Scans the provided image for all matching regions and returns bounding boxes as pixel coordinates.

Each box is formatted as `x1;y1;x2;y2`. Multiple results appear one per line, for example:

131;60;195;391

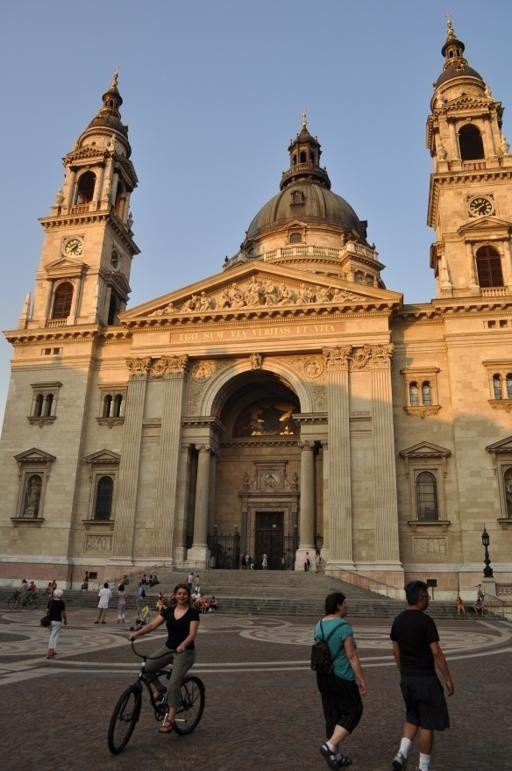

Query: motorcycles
8;588;40;610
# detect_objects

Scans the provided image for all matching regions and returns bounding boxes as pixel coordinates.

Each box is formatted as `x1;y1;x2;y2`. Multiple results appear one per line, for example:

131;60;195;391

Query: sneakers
94;621;106;624
391;753;409;771
415;767;434;771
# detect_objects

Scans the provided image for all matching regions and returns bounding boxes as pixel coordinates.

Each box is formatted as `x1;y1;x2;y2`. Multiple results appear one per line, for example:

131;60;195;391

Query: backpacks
309;619;350;674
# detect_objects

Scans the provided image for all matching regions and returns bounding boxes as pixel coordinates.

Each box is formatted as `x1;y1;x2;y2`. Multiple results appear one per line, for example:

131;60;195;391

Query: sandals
337;754;352;767
153;686;168;703
320;743;341;771
159;719;176;735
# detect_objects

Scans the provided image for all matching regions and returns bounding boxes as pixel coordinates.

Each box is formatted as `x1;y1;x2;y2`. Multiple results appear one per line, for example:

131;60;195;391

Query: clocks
467;194;495;217
110;248;122;271
65;239;82;256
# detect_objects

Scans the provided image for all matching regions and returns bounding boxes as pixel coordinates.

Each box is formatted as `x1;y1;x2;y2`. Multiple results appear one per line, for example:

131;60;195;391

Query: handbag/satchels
40;615;52;628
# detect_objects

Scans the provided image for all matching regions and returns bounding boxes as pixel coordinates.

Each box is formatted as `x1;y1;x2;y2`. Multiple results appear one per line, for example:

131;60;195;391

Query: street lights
482;522;493;577
293;523;298;532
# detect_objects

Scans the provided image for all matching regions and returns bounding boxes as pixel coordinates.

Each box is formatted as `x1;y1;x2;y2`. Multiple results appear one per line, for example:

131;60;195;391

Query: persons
315;551;321;573
147;275;368;317
13;579;67;659
94;573;218;733
313;592;367;771
304;552;309;572
226;548;285;570
456;584;484;617
390;581;454;771
80;571;88;592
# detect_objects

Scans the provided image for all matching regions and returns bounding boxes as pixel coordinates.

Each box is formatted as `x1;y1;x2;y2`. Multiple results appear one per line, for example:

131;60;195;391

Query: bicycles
107;636;206;755
